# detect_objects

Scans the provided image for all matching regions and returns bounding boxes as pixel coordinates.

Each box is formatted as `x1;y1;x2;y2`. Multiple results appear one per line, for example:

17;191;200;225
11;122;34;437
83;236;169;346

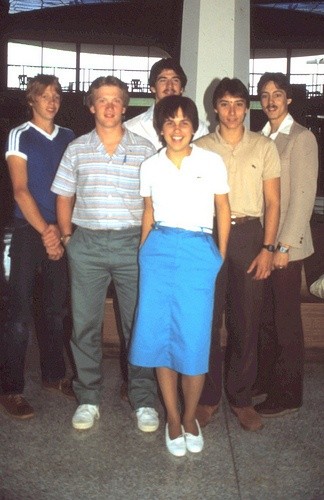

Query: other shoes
231;400;264;432
44;373;74;399
254;393;303;418
165;418;205;457
195;402;221;427
1;392;36;419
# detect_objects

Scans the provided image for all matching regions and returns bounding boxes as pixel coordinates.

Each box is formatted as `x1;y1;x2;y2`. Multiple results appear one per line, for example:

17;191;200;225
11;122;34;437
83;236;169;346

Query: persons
126;95;231;457
0;75;78;419
50;75;161;432
122;59;210;152
192;76;281;431
250;73;319;417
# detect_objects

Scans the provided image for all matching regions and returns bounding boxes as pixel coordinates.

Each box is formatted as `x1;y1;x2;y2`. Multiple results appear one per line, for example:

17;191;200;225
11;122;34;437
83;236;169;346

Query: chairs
131;79;143;93
63;83;74;93
17;75;27;90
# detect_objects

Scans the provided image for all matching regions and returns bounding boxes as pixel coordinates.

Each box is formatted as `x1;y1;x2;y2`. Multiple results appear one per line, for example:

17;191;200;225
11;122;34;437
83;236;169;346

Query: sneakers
72;403;100;430
136;406;160;433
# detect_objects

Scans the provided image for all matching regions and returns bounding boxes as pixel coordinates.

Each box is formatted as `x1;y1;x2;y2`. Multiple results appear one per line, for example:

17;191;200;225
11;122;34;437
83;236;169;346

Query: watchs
277;244;289;254
262;244;275;252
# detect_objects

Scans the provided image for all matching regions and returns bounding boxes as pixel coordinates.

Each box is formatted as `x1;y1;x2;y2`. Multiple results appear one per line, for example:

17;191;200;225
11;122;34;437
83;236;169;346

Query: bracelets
61;233;73;240
